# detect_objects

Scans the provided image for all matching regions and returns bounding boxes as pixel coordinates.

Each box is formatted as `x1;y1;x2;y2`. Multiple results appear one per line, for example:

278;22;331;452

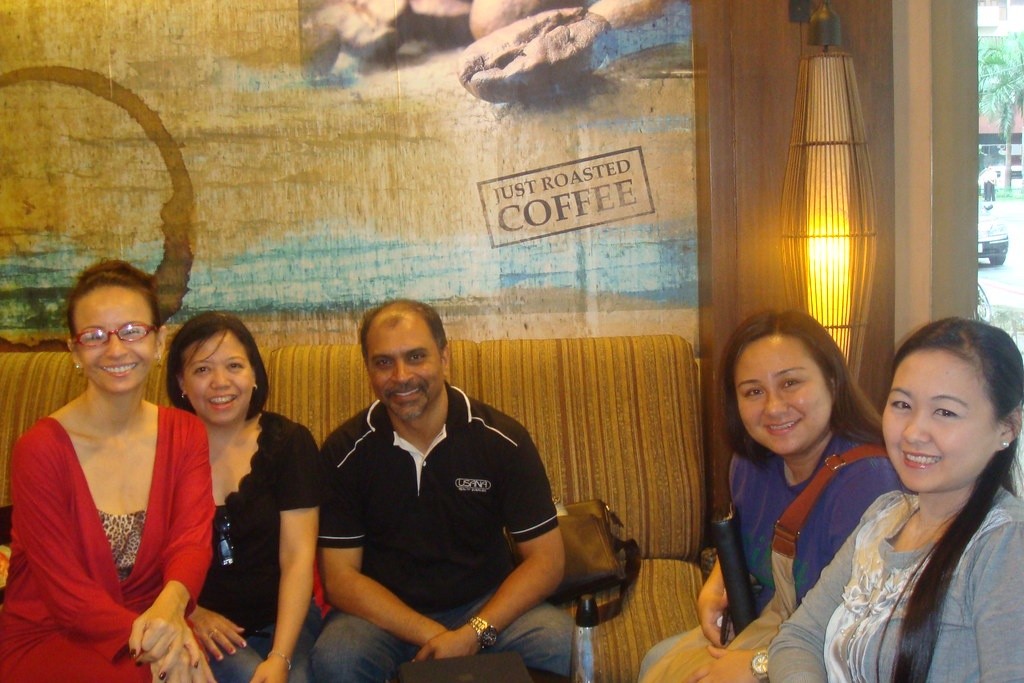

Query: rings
209;630;217;636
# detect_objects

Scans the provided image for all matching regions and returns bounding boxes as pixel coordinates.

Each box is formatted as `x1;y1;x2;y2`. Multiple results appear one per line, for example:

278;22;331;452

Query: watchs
469;617;497;650
752;647;769;683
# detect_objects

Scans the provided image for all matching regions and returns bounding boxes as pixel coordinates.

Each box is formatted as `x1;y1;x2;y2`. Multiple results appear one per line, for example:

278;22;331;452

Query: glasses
218;512;235;560
72;323;158;347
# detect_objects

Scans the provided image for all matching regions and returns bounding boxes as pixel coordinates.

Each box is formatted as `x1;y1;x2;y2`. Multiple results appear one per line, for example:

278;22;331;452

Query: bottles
570;594;605;683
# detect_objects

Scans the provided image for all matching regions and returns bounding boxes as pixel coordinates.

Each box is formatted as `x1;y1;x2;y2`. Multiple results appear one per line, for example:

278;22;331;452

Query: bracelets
268;651;292;670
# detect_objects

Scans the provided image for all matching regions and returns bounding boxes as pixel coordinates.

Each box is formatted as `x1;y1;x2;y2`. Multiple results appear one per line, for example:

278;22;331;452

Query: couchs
0;334;708;683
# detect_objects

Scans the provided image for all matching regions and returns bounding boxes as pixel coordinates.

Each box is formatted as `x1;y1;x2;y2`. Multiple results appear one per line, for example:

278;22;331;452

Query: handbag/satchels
548;498;641;628
637;593;797;683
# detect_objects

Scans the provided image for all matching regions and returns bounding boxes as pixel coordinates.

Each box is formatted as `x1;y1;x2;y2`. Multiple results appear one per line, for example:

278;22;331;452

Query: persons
983;170;1000;201
769;317;1024;683
167;311;321;683
0;260;216;683
314;299;574;683
640;305;918;683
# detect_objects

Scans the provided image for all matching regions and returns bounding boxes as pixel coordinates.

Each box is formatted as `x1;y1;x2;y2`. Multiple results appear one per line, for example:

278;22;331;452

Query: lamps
779;0;878;380
